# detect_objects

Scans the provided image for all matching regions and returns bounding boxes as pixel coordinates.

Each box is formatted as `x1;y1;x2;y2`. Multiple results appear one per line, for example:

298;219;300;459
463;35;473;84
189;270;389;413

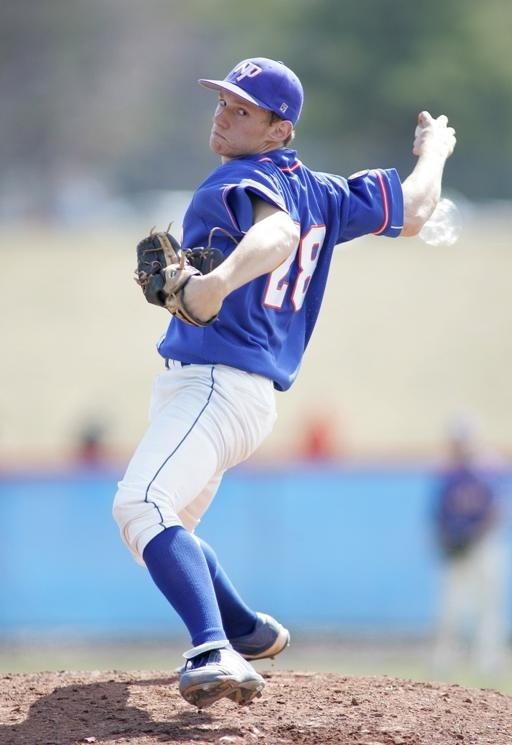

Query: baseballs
415;115;438;140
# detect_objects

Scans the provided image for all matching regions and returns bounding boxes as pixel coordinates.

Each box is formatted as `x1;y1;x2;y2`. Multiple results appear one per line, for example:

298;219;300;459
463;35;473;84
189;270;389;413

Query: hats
197;57;305;129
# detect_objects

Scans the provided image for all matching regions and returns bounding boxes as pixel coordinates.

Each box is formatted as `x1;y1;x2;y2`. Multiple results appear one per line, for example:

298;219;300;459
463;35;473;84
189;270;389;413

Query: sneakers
177;641;266;709
228;611;291;662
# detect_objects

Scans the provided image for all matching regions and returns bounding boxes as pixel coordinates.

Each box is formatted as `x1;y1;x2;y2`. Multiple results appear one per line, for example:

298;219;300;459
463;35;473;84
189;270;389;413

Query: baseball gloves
135;226;237;328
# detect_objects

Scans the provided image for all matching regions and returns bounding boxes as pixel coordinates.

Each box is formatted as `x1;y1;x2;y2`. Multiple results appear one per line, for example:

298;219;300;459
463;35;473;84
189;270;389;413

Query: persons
433;442;501;682
111;55;456;708
